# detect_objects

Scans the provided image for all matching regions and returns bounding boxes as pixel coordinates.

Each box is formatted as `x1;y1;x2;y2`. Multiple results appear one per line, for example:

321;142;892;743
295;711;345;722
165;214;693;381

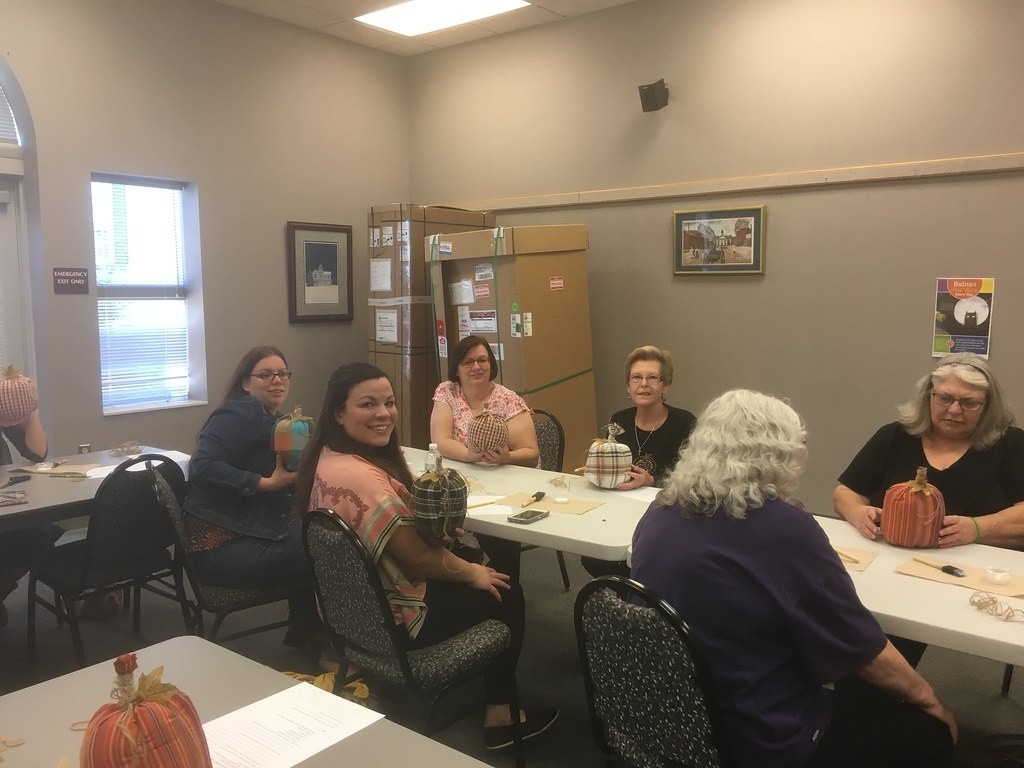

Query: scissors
0;475;32;490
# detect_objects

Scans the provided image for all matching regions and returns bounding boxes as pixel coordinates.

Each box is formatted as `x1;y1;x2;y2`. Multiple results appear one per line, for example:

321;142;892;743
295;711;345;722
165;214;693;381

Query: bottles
424;444;442;473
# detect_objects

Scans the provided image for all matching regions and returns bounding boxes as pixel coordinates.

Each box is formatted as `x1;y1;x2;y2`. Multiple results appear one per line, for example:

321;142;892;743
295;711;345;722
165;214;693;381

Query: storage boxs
411;225;600;472
362;204;497;453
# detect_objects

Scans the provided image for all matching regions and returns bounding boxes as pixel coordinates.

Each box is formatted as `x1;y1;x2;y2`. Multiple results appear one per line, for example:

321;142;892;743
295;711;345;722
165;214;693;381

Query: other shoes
483;708;559;750
282;630;328;651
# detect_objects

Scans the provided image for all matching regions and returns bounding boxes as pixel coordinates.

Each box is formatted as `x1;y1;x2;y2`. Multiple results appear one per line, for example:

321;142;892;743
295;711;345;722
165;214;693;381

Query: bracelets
969;517;981;544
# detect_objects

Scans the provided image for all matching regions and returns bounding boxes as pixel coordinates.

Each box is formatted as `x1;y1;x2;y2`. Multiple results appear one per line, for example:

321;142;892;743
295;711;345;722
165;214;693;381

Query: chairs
28;411;1015;768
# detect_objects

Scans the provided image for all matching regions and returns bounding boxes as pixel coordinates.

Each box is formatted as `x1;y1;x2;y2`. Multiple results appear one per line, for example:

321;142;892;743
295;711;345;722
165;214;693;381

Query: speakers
638;78;669;112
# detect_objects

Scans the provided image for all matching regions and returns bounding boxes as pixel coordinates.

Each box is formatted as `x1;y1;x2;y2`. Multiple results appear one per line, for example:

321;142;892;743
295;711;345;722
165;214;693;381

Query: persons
429;335;542;579
629;386;961;767
574;344;697;579
288;360;559;751
181;342;331;650
833;348;1024;667
0;363;47;626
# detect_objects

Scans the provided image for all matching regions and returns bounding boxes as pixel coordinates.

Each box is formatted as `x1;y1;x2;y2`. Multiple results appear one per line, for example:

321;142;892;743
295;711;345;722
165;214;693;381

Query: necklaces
634;413;663;456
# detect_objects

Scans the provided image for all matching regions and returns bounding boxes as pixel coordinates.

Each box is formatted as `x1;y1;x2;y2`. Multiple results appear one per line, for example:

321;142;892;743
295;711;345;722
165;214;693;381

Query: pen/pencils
574;466;585;471
467;502;495;508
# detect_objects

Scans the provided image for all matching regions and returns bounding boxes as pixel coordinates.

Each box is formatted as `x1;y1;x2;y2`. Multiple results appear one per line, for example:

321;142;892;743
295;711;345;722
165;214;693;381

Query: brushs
913;556;966;576
521;492;546;508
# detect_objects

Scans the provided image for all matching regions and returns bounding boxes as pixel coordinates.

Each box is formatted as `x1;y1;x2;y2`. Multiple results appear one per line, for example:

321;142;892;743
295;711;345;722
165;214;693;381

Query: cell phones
508;508;549;525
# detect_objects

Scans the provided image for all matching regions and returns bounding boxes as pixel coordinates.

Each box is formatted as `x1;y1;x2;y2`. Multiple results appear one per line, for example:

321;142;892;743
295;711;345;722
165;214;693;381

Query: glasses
459;358;489;368
251;371;291;380
932;388;987;411
629;373;663;384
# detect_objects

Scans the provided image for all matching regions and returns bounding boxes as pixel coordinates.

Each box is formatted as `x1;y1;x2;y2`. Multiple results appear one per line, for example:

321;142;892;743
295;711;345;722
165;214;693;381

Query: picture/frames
673;206;767;275
287;219;355;323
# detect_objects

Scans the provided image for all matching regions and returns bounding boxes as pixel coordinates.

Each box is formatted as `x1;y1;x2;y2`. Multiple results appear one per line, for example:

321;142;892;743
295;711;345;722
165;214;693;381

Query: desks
0;636;494;768
398;443;664;562
628;512;1024;659
0;437;195;537
